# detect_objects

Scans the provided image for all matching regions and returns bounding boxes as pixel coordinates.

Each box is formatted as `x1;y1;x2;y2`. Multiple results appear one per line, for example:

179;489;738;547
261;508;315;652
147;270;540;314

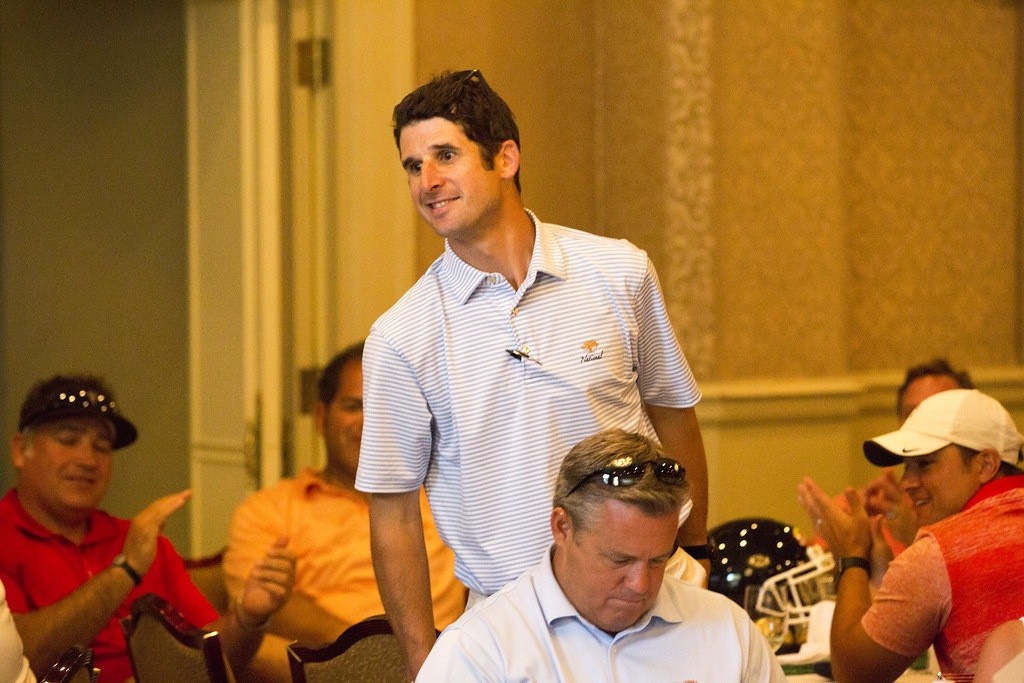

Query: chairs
40;593;443;683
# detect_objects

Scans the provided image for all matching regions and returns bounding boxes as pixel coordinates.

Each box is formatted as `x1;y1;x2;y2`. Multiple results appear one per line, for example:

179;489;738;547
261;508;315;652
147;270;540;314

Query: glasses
559;457;686;508
453;69;511;137
41;376;116;413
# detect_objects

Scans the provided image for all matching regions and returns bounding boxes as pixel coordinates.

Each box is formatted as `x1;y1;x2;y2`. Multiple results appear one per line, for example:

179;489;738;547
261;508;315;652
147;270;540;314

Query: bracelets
236;598;274;633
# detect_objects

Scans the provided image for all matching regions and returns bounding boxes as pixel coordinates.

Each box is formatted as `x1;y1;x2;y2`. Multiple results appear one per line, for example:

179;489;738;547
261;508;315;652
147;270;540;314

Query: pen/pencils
506;349;542;366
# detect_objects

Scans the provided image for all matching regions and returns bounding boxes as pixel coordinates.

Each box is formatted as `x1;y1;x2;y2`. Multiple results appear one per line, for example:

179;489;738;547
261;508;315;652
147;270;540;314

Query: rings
816;519;822;531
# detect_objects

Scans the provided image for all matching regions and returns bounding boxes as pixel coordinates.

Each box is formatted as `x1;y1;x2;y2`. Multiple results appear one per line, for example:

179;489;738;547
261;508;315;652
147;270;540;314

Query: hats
18;375;137;449
862;388;1024;465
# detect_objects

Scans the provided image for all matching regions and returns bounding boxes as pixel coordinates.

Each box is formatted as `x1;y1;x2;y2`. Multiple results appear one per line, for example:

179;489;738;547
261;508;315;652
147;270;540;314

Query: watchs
833;557;871;589
683;536;719;559
113;555;141;584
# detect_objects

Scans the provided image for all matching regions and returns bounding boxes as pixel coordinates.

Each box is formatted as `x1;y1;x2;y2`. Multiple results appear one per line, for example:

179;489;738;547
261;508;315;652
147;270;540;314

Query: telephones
706;518;817;622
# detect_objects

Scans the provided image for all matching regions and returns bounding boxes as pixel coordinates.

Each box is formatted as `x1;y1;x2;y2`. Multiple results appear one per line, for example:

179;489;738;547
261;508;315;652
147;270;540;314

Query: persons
355;71;710;676
798;362;1024;683
414;427;787;683
0;369;296;683
225;338;470;683
